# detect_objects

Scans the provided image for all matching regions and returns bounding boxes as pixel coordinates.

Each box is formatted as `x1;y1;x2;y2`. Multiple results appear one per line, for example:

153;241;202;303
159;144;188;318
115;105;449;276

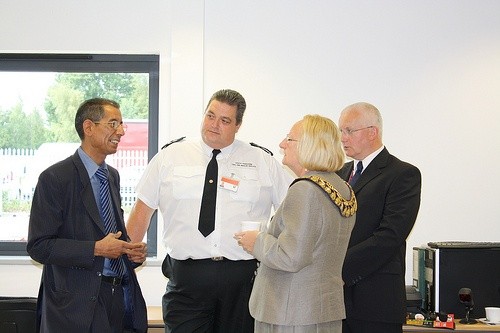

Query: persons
232;114;358;333
126;89;296;333
333;101;423;333
26;97;149;333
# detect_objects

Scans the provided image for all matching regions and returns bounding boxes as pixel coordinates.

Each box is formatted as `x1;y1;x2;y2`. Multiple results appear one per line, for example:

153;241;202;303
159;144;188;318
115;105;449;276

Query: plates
479;318;500;324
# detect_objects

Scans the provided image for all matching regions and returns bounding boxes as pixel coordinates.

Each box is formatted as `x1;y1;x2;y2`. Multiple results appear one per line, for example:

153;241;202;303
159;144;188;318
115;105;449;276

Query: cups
242;221;260;234
485;307;500;323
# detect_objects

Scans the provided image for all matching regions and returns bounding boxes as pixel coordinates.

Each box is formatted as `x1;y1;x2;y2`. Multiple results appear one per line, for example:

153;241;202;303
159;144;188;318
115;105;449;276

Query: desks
402;318;500;333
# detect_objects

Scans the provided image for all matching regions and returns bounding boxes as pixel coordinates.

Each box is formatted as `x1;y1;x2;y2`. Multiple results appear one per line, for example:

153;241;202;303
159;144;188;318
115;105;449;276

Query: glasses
286;132;300;143
93;121;128;132
340;126;373;136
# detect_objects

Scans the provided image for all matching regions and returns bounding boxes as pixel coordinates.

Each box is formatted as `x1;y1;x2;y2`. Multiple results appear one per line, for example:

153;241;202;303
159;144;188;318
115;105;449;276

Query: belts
102;276;128;287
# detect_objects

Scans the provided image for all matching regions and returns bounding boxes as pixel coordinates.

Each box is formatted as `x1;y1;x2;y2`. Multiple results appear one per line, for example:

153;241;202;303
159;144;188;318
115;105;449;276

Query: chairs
0;298;39;333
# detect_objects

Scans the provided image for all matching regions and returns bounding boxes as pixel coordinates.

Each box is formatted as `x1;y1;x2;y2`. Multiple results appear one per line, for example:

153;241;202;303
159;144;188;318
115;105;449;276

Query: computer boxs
424;247;500;320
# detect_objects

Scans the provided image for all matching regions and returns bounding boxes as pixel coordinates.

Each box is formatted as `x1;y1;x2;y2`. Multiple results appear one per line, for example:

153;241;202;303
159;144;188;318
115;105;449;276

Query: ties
198;148;222;238
97;166;125;279
350;160;363;187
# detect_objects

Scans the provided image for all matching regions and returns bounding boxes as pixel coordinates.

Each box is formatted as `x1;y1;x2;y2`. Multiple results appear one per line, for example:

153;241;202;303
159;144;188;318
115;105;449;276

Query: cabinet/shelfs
414;241;500;319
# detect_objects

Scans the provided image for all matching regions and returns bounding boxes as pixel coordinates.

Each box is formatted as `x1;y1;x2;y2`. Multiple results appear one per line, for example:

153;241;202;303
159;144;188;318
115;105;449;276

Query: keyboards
427;240;500;248
